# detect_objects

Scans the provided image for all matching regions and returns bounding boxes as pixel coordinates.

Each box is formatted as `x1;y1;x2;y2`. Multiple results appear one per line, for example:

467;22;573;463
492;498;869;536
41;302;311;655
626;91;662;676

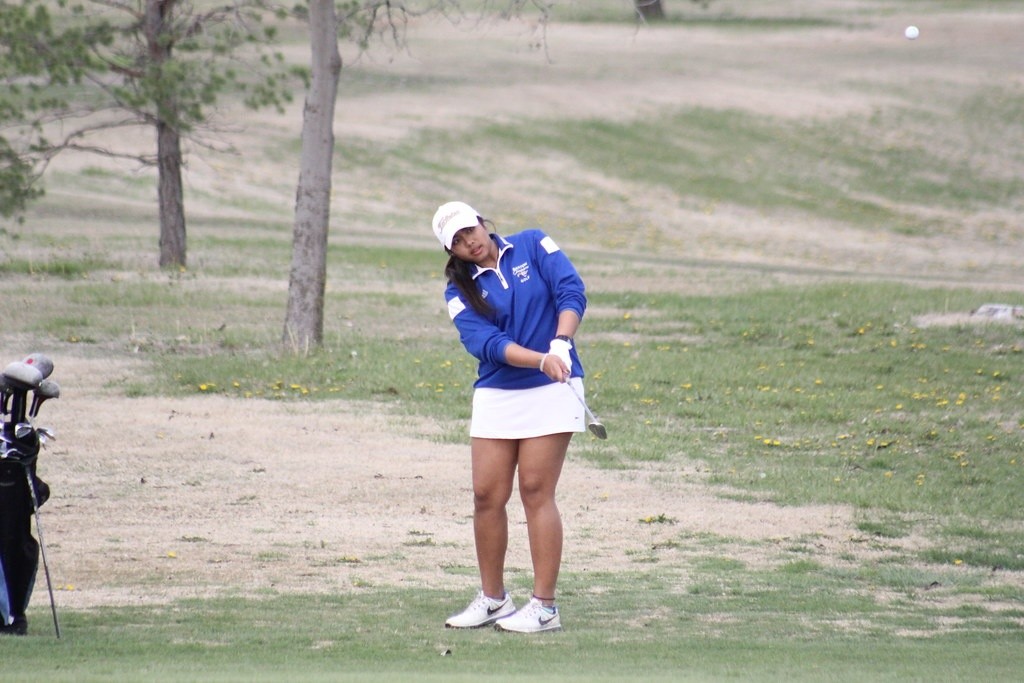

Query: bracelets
555;335;573;345
539;353;548;371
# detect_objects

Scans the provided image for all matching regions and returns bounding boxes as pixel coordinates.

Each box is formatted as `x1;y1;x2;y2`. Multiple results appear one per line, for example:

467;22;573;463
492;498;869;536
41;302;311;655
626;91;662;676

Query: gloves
549;338;573;382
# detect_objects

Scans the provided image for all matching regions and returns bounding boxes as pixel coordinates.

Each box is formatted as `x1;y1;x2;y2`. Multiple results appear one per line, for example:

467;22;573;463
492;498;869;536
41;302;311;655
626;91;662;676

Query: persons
432;201;587;632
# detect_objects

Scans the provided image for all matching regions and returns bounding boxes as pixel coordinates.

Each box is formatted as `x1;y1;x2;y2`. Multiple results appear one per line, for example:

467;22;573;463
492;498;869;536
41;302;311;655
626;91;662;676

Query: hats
432;201;481;252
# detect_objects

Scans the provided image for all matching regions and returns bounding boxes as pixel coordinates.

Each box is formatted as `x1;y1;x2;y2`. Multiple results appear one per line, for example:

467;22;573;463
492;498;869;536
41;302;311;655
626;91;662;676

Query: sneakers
444;590;516;629
494;599;561;633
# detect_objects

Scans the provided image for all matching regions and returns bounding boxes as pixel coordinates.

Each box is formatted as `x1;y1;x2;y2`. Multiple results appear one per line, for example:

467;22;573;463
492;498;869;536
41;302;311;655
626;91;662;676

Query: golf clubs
561;377;607;439
1;354;61;458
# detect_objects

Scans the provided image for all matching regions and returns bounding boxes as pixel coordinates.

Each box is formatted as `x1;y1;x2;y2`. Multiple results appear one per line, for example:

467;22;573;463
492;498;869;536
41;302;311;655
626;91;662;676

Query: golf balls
905;26;919;39
